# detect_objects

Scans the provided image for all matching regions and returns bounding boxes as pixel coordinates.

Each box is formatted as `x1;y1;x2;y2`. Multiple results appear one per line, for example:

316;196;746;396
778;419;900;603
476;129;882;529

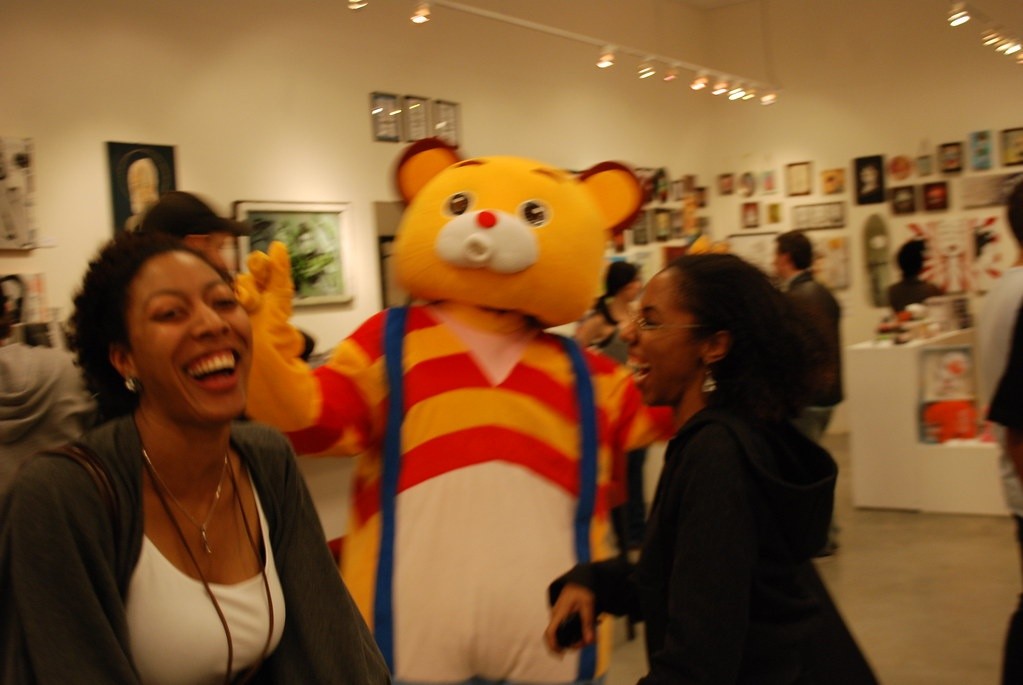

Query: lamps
347;0;369;9
596;45;777;105
410;2;431;25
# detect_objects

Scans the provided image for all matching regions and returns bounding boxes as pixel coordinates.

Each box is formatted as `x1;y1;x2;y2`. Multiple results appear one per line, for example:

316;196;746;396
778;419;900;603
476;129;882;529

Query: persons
773;225;841;444
0;300;85;507
542;256;884;685
571;260;648;552
0;228;394;685
888;238;942;311
134;186;266;274
969;176;1023;683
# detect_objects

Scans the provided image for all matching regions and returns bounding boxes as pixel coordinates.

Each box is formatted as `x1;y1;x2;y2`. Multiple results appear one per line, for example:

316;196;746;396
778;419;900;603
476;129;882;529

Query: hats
131;191;256;246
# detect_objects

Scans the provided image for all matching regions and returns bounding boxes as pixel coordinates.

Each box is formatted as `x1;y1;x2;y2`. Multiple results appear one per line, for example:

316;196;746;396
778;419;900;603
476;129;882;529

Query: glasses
628;304;711;329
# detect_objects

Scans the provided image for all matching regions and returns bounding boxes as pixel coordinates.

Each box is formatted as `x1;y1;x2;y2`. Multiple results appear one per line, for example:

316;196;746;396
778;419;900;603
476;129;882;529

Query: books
927;295;974;328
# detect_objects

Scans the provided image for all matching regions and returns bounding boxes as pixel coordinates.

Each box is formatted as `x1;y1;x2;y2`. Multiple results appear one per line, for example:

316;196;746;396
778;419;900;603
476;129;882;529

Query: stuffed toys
234;138;665;684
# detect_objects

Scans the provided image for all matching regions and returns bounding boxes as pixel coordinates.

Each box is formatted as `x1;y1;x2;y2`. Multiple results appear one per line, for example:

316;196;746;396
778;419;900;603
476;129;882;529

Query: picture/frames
403;94;431;143
233;199;356;306
369;93;401;142
1002;127;1023;165
785;161;810;197
0;139;37;251
433;99;460;148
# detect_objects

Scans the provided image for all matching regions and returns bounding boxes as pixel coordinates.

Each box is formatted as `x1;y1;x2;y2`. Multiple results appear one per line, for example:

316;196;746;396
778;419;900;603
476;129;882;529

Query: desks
848;325;1007;514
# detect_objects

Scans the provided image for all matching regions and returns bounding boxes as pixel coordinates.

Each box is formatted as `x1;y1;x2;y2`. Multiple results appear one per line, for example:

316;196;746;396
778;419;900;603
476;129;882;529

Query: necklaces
139;441;228;554
